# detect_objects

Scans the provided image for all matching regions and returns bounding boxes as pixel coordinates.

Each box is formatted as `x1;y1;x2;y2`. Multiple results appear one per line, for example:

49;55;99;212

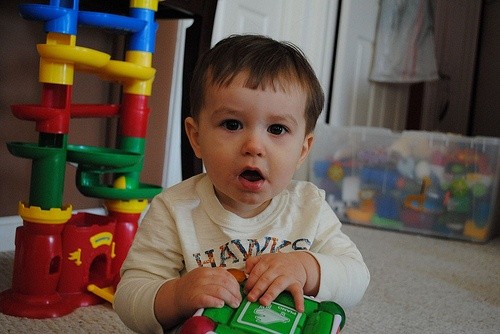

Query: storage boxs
307;122;500;246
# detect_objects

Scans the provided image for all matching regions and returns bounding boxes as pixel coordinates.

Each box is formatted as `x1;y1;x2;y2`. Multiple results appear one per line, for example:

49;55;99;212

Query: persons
113;35;369;333
